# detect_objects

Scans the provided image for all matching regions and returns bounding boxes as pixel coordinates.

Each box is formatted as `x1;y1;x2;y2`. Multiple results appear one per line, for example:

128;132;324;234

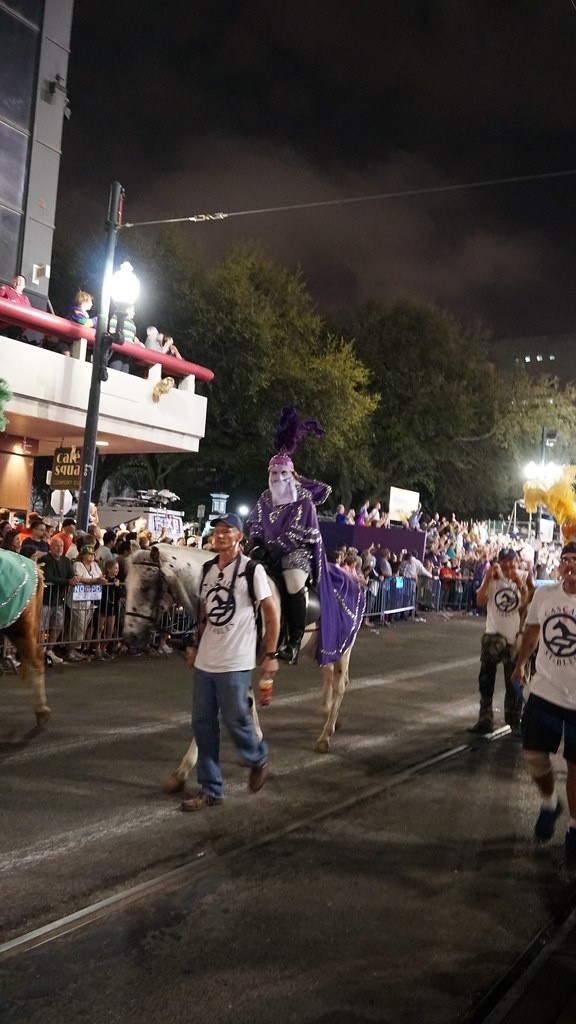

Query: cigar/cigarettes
569;571;571;575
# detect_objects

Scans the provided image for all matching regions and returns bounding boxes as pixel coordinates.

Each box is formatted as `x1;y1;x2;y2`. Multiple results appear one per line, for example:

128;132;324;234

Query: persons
510;541;576;861
420;511;564;614
469;547;528;731
0;503;247;668
332;543;435;628
242;453;322;659
180;513;280;808
335;497;423;531
0;274;184;389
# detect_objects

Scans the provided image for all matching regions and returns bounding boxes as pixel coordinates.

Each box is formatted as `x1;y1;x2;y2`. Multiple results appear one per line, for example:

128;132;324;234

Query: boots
278;587;306;666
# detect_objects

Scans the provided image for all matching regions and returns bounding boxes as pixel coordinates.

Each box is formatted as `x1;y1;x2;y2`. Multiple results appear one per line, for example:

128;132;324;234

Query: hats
81;545;95;554
268;406;332;506
499;548;516;560
63;519;76;527
561;541;576;556
210;513;243;532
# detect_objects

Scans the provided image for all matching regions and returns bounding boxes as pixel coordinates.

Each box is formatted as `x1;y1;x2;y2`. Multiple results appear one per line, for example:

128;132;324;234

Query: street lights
520;425;560;564
238;505;250;524
75;178;141;530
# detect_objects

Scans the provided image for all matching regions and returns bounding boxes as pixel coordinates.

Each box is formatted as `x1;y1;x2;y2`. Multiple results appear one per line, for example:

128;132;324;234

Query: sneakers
249;762;267;793
182;790;222;812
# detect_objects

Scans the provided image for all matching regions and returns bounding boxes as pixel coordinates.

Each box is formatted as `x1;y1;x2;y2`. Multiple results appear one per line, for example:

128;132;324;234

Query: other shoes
506;720;522;737
565;826;576;863
7;642;173;668
534;801;561;840
467;720;492;732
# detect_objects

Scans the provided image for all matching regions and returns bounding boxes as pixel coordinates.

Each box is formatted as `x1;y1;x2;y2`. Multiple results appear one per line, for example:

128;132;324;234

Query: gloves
243;541;270;561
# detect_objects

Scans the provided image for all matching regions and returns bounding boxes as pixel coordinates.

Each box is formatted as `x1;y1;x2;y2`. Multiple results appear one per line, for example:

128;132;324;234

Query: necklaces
217;556;239;580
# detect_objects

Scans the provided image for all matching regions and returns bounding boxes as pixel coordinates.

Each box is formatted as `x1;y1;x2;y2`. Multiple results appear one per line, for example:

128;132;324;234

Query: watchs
266;652;279;659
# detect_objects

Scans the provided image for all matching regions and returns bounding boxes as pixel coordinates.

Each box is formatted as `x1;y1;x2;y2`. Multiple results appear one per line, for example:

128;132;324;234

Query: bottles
258;674;274;705
492;561;501;579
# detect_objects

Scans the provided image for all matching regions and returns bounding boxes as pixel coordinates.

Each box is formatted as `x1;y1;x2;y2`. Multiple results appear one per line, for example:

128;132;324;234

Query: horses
121;543;368;795
0;545;52;730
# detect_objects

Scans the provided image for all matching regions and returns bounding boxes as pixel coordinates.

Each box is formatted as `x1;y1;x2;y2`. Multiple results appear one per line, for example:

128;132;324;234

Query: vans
94;488;186;544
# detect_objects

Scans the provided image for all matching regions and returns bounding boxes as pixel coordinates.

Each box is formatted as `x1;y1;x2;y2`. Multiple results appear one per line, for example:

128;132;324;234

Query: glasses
561;556;576;565
